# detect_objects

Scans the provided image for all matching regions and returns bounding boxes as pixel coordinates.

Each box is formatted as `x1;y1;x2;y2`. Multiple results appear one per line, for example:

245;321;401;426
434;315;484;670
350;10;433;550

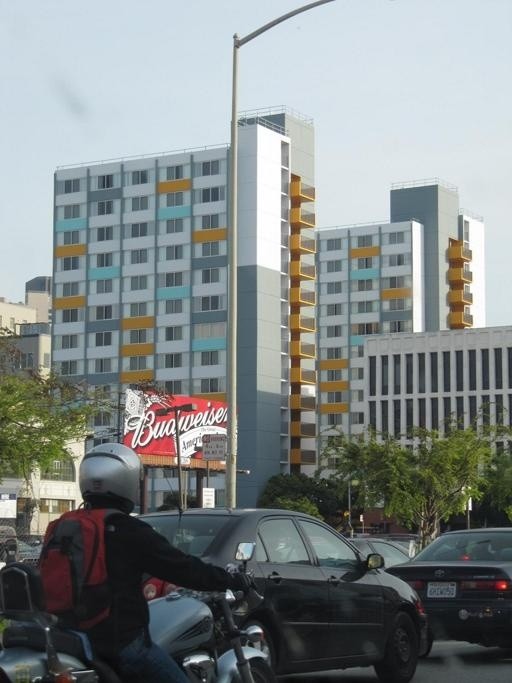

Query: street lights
154;403;198;510
346;473;359;525
133;466;428;681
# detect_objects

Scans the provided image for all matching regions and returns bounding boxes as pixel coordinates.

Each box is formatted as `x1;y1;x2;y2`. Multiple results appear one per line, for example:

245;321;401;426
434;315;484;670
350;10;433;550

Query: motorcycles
0;540;274;682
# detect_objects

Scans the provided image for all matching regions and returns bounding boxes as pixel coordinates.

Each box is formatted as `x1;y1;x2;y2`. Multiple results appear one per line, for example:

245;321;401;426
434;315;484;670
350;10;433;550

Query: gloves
230;572;259;593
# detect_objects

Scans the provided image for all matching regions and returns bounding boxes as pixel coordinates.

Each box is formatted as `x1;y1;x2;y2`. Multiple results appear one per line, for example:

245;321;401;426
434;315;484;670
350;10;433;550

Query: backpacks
40;507;128;631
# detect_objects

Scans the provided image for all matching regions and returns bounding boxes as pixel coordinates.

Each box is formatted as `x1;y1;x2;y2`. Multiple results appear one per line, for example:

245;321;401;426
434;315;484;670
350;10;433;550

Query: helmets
79;443;145;513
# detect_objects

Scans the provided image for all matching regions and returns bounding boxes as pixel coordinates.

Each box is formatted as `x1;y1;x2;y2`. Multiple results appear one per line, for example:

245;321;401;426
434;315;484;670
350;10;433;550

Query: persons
36;441;255;681
